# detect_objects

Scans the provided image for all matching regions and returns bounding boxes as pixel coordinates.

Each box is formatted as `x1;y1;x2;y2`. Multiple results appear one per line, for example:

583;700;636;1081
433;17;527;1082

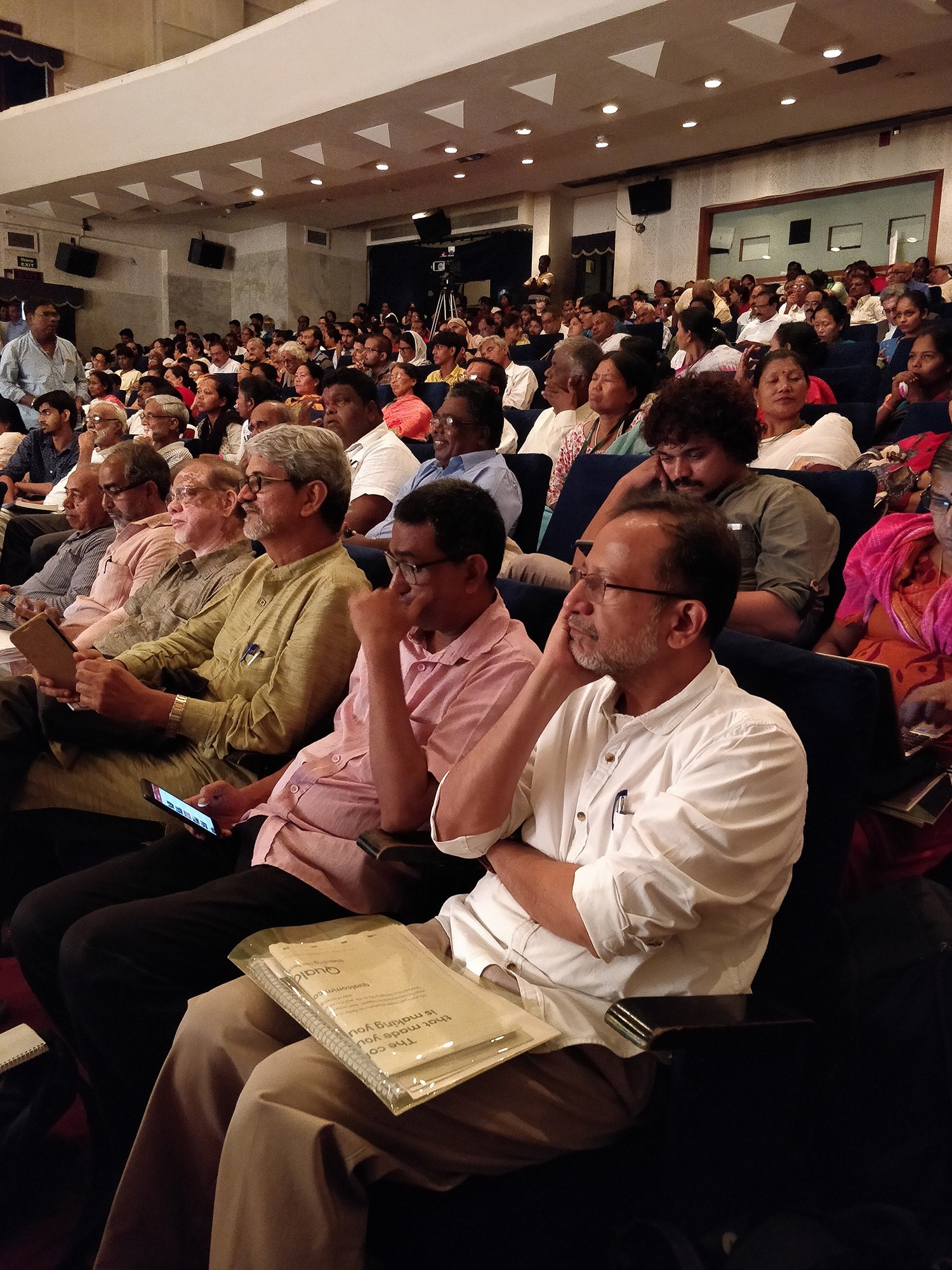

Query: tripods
429;278;458;342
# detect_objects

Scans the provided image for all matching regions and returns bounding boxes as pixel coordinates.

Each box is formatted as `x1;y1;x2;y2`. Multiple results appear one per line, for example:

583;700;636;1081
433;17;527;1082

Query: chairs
217;318;952;1270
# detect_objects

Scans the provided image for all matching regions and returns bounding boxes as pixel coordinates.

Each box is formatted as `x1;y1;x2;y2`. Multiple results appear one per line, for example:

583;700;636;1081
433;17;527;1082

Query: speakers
187;237;226;270
411;208;451;241
54;242;99;278
628;179;672;215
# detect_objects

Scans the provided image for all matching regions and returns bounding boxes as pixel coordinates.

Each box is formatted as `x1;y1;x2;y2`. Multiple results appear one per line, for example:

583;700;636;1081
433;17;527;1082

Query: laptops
710;626;952;760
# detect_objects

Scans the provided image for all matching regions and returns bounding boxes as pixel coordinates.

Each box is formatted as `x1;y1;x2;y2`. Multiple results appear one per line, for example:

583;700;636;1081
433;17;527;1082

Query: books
0;1023;50;1074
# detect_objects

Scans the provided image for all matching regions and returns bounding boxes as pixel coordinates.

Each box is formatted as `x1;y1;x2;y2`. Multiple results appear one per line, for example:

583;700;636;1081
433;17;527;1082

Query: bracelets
164;694;188;739
881;402;896;413
30;397;39;409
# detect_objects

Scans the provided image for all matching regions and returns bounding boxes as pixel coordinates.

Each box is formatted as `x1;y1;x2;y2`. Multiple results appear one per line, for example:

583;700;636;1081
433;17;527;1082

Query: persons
96;489;809;1270
521;255;555;311
0;297;92;436
0;256;952;1257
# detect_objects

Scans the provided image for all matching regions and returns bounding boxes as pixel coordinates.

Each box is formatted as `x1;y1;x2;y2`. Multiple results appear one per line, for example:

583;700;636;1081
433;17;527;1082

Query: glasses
267;350;278;355
390;374;413;380
99;477;157;501
457;374;497;386
921;483;952;514
27;310;60;320
384;551;477;585
882;307;900;316
238;473;310;494
364;347;383;353
890;269;910;276
95;358;107;365
239;367;251;371
754;303;774;311
397;346;414;353
568;565;711;605
84;417;122;424
792;285;808;289
411;315;419;318
165;484;237;507
152;346;165;349
428;415;487;432
248;421;286;432
140;413;180;423
579;311;594;316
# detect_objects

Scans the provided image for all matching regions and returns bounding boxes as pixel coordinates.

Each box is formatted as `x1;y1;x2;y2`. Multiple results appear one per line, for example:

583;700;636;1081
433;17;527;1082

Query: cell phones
1;600;30;621
139;778;224;840
575;539;594;558
9;613;81;697
